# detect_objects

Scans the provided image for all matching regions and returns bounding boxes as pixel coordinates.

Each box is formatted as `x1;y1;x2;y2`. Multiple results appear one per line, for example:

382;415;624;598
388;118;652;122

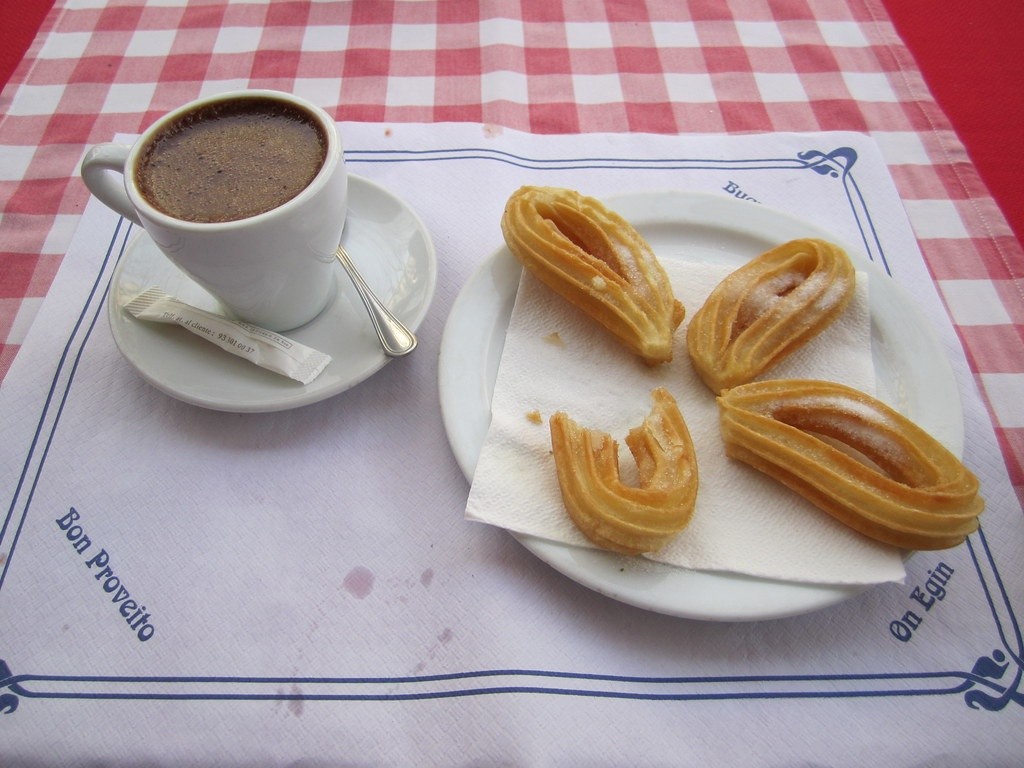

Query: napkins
462;255;907;586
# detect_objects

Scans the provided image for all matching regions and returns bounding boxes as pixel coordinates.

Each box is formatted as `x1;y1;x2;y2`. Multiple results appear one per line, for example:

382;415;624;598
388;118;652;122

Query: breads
500;185;688;368
549;386;698;555
716;378;986;550
686;238;857;396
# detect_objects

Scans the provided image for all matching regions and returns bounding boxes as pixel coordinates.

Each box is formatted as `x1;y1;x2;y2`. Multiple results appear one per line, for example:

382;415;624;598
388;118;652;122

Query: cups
80;89;347;332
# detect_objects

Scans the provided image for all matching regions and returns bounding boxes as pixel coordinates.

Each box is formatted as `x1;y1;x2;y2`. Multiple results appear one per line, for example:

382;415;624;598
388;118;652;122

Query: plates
107;173;437;413
437;192;964;624
